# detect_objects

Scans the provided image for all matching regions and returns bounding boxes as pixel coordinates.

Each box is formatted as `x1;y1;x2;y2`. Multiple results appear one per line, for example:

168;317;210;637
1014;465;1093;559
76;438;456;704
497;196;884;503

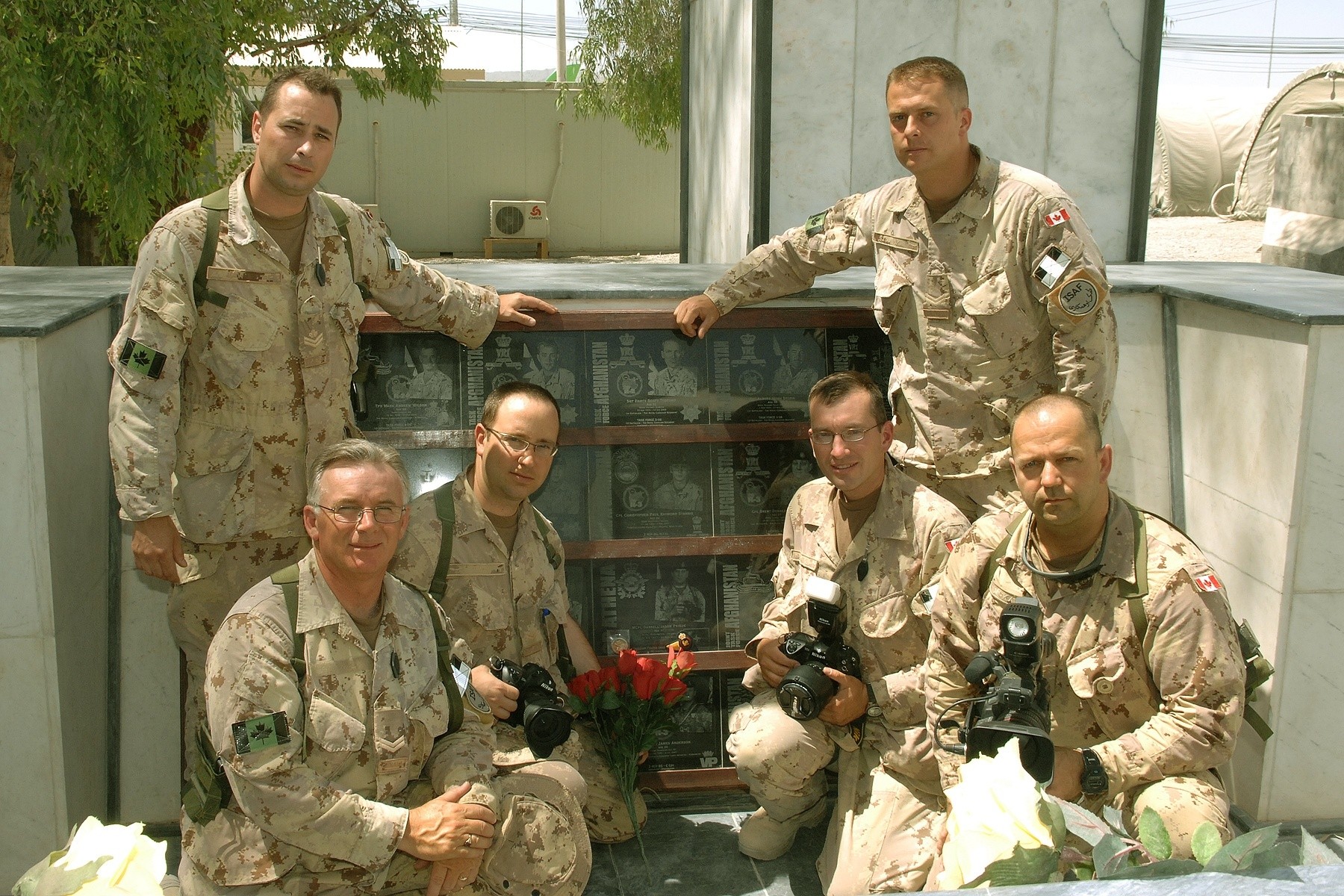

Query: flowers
567;633;698;889
10;813;180;895
937;732;1344;890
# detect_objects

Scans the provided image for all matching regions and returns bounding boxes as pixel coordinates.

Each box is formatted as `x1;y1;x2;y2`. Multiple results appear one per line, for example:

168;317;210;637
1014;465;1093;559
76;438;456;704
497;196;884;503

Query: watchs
1081;748;1108;803
866;682;882;718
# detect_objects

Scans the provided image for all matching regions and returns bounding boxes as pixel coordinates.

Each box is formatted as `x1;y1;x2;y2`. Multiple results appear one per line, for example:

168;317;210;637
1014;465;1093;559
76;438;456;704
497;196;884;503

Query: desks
482;237;549;259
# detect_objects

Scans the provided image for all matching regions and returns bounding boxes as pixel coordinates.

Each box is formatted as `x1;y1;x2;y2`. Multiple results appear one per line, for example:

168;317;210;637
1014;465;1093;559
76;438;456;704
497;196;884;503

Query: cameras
776;575;859;720
491;658;573;760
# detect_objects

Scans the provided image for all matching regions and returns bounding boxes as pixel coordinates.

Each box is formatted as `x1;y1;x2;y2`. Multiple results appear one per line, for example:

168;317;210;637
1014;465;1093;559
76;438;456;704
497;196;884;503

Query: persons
387;381;647;845
673;57;1118;527
724;369;972;896
925;397;1244;896
407;339;818;734
180;439;591;896
108;68;560;853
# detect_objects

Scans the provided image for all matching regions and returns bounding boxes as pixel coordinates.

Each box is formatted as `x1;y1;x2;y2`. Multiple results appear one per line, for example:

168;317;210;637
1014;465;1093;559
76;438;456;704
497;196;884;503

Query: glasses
315;504;405;524
809;420;887;444
484;427;559;459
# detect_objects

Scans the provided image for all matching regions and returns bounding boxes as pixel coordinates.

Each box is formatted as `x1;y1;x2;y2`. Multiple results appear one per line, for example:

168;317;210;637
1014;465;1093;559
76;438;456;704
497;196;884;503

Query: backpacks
1209;62;1344;222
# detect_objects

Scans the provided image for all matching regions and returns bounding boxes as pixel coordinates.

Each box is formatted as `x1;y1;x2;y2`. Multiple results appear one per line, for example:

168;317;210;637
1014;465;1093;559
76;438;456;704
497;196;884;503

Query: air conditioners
489;200;550;239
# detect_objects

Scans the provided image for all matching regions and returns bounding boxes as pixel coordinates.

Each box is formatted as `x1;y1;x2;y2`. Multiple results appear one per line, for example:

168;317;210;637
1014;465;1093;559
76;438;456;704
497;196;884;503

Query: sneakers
738;790;828;860
178;816;219;895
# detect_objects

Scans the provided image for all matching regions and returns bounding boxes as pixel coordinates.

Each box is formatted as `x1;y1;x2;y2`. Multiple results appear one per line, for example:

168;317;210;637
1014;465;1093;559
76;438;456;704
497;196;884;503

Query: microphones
964;649;999;684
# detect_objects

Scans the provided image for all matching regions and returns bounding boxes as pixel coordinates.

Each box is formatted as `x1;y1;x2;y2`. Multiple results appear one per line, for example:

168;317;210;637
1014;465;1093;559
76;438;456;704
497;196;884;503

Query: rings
458;876;467;880
465;833;472;848
